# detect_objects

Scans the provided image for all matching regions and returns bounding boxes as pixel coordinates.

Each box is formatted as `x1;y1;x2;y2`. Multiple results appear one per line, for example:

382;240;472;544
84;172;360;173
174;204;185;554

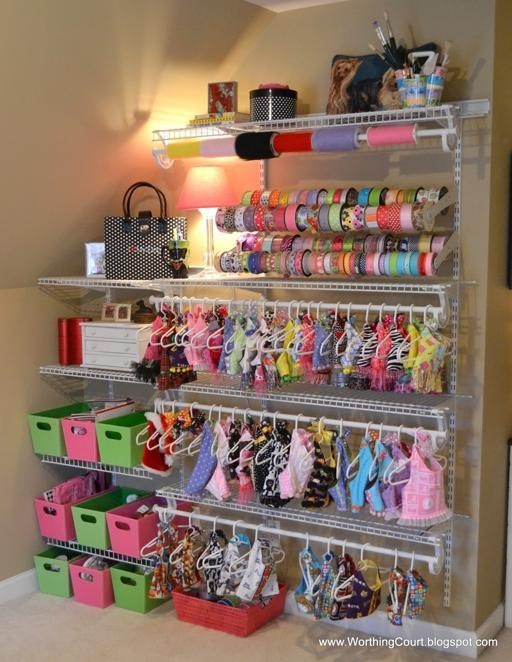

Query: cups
161;240;191;278
397;73;445;109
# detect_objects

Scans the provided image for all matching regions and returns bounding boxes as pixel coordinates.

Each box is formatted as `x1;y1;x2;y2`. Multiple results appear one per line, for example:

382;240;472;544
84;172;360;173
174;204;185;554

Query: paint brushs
367;10;404;70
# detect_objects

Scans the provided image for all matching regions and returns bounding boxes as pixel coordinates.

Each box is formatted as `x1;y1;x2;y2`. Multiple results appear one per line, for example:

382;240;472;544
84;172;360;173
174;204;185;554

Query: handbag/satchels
104;181;187;280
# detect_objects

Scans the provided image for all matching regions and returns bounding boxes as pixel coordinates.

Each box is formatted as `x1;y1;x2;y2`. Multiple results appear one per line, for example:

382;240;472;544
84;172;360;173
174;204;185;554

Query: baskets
170;583;288;638
